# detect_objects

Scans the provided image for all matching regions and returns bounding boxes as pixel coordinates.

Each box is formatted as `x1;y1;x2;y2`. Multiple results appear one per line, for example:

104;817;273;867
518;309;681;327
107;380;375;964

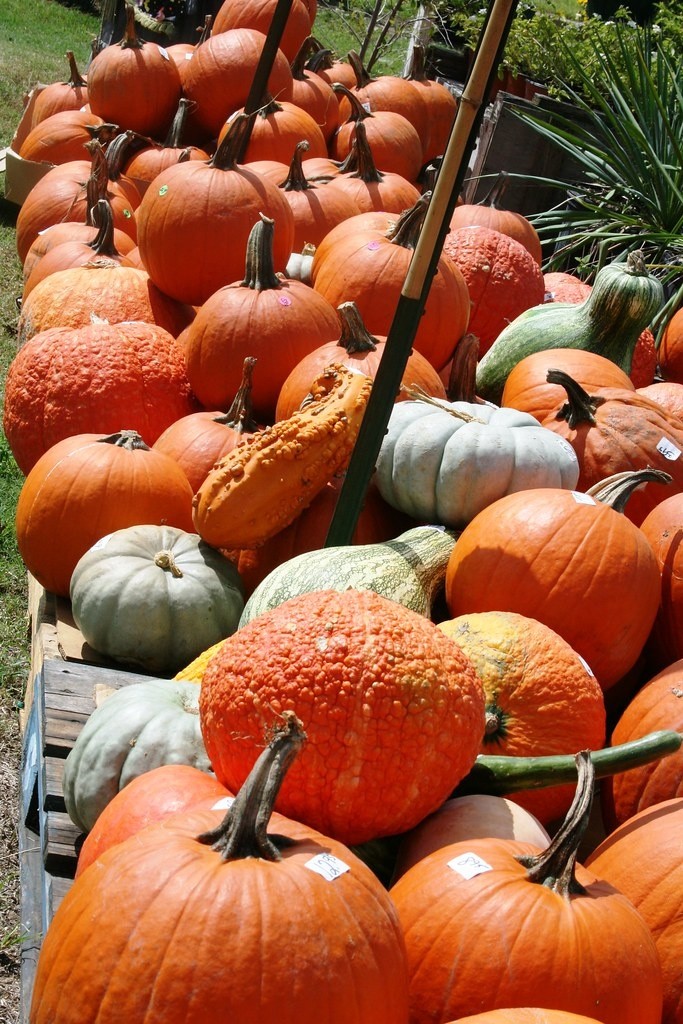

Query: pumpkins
14;1;682;1024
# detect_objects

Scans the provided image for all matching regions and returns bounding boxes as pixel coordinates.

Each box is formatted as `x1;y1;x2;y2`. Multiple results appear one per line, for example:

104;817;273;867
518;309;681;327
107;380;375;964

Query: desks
16;659;164;1024
19;570;141;745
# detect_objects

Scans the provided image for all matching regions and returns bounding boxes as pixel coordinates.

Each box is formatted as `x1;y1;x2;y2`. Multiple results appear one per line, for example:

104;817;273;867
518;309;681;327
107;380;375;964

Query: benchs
406;45;624;277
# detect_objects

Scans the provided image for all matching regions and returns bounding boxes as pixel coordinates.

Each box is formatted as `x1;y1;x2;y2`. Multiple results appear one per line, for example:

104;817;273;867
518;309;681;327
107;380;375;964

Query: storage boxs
4;83;59;205
